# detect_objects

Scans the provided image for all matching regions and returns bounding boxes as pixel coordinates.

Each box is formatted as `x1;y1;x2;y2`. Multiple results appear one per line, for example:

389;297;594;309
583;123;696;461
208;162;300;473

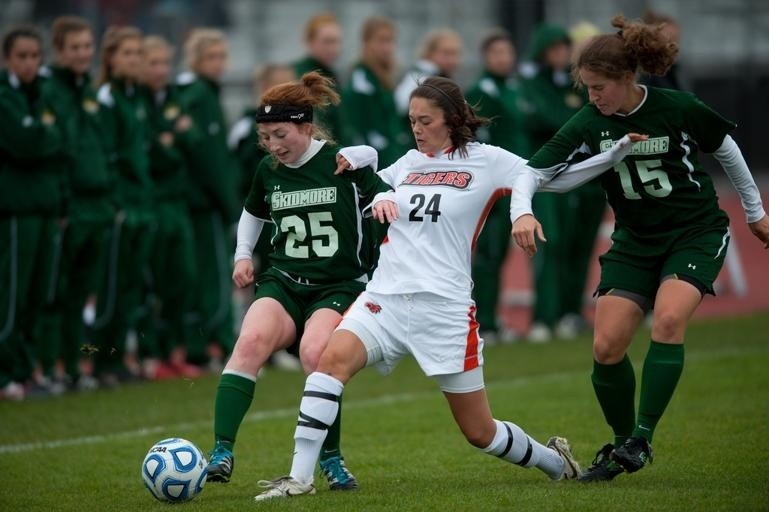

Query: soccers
143;437;209;504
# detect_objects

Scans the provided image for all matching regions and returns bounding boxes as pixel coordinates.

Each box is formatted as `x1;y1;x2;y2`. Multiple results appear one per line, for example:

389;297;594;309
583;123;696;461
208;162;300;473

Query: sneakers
255;477;316;500
206;448;234;482
546;435;653;481
320;456;357;490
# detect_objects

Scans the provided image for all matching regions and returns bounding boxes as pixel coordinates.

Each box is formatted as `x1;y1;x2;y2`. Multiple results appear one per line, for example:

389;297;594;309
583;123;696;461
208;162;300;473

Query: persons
507;13;769;482
0;13;610;405
253;74;651;504
636;10;686;90
205;68;398;492
